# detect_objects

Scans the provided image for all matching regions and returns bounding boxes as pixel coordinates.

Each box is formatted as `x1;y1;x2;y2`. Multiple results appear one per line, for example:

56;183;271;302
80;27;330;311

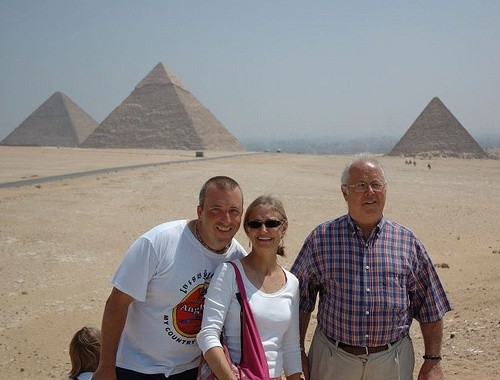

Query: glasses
245;219;285;228
343;179;387;193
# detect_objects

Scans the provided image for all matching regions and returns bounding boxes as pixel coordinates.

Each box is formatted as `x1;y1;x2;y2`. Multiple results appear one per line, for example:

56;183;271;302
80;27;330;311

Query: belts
324;333;394;355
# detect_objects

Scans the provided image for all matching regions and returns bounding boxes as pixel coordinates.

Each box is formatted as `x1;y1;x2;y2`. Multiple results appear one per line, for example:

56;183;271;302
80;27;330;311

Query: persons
68;176;253;380
284;152;451;380
196;195;306;380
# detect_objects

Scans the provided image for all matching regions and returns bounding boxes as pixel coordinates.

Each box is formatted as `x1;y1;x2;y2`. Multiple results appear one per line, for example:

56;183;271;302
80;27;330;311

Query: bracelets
301;346;305;351
422;354;441;360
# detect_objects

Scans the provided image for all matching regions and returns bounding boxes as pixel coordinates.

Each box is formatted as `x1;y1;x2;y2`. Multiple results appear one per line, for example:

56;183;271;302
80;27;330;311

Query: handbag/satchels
196;261;269;380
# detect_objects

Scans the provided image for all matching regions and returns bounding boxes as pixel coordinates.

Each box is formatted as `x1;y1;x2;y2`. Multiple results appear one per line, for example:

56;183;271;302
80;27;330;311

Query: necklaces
247;258;268;293
195;220;231;252
